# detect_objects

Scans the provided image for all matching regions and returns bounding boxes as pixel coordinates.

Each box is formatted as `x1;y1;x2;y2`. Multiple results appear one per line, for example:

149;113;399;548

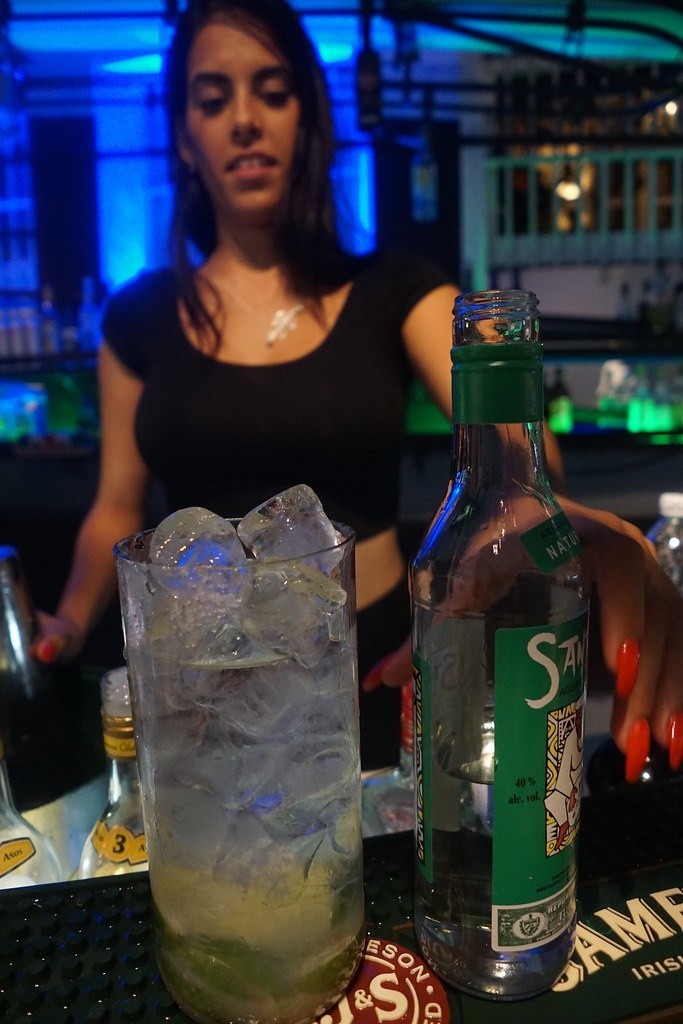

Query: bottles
80;669;150;876
615;283;635;321
0;294;36;359
0;750;62;888
640;278;654;334
406;292;589;998
40;288;62;355
648;491;683;596
78;277;102;355
0;546;45;694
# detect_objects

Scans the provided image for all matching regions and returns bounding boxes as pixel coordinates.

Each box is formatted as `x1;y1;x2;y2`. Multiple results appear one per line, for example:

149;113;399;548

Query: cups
113;521;364;1024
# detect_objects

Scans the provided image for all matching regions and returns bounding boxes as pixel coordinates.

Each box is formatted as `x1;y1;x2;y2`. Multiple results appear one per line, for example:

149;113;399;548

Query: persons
36;0;683;785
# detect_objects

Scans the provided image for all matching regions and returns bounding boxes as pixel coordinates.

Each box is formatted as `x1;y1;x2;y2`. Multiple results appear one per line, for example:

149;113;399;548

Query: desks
3;766;683;1023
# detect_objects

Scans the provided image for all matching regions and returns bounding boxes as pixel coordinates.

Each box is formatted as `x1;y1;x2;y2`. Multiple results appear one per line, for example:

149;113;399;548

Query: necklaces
209;260;304;343
